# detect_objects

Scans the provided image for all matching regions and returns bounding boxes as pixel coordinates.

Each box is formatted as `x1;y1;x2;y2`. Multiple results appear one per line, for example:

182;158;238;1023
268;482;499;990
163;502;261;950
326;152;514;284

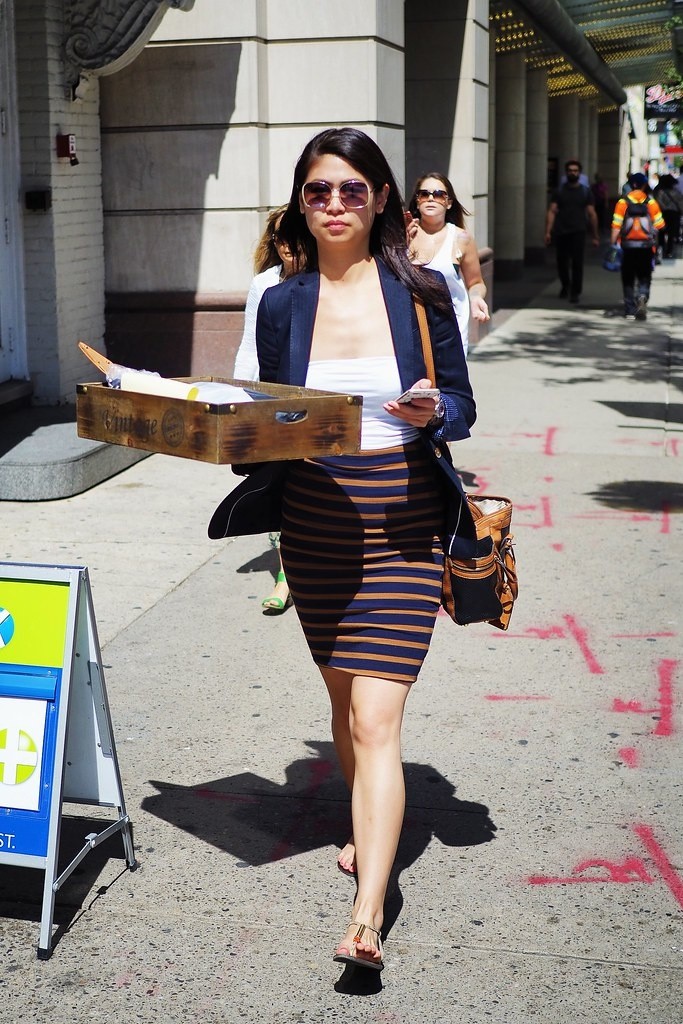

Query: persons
405;172;491;362
608;173;666;320
232;203;292;609
621;157;683;270
591;172;608;234
208;128;477;971
544;161;601;304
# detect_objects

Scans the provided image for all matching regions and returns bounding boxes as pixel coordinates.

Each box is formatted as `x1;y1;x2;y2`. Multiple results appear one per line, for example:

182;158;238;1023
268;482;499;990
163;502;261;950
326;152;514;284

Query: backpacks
622;194;655;240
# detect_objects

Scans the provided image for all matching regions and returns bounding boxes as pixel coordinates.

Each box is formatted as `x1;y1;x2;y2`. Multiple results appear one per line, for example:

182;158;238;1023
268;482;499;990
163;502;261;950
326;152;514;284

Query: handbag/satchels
443;494;519;630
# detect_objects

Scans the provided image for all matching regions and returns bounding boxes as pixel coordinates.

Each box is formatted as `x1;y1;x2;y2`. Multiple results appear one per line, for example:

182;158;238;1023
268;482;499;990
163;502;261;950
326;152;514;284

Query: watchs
430;396;445;425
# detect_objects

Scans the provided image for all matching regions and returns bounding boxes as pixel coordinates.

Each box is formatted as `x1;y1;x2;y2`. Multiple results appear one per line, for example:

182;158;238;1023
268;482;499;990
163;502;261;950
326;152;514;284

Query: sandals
337;837;361;877
332;921;385;971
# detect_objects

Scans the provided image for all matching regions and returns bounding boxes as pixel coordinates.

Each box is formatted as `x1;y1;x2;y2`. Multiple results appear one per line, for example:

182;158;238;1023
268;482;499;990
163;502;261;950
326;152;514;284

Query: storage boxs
76;374;365;466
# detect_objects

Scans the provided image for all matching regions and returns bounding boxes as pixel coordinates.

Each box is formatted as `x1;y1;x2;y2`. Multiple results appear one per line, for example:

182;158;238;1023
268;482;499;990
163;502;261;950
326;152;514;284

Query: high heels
263;571;293;611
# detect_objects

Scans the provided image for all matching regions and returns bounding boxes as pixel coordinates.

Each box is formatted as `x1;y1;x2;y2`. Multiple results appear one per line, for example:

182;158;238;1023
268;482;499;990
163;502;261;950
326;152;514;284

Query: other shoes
624;313;635;319
636;300;645;320
571;291;579;303
560;285;569;297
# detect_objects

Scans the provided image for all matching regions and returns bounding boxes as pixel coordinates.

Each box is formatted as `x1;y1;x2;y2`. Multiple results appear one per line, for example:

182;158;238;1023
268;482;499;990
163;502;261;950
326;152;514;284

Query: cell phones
395;388;441;404
403;211;414;227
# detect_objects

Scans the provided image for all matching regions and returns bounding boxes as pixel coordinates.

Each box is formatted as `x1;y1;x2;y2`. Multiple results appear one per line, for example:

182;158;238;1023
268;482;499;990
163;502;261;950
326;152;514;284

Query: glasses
416;189;450;203
272;231;291;246
301;180;378;211
